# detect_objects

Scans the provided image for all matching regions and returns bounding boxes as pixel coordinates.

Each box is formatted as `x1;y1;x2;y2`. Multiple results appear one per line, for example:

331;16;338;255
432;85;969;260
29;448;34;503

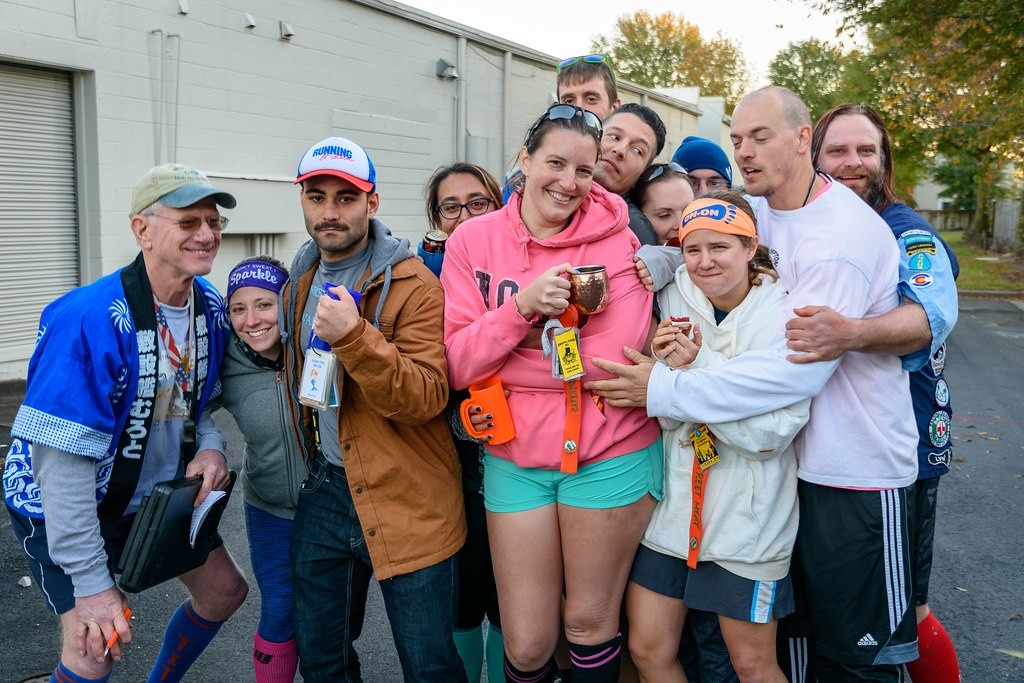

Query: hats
295;137;376;193
128;163;237;219
672;136;732;188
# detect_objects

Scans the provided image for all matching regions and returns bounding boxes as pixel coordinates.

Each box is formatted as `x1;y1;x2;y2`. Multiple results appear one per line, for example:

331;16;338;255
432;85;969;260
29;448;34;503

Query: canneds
422;230;448;254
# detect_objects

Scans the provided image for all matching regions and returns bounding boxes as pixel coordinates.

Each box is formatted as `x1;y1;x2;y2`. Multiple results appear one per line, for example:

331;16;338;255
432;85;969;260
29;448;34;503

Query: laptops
116;469;235;593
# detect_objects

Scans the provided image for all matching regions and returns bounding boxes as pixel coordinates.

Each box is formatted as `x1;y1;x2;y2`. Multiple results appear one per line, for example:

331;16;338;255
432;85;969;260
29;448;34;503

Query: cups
459;376;516;445
569;264;610;315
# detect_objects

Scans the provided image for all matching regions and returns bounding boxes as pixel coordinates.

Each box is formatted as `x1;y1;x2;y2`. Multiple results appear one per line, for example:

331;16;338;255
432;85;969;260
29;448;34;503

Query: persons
497;54;964;683
0;164;249;683
276;136;471;681
214;254;317;683
629;189;815;682
441;103;667;682
418;163;504;682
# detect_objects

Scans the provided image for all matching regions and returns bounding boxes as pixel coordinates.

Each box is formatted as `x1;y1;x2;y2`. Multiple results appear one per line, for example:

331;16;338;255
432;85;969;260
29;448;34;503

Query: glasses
556;54;615;84
435;198;494;219
144;214;231;232
690;178;729;190
526;104;603;146
638;162;689;182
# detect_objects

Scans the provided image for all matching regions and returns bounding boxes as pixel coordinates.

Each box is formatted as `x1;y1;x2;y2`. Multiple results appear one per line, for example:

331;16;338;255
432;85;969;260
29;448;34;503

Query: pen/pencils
104;607;132;657
